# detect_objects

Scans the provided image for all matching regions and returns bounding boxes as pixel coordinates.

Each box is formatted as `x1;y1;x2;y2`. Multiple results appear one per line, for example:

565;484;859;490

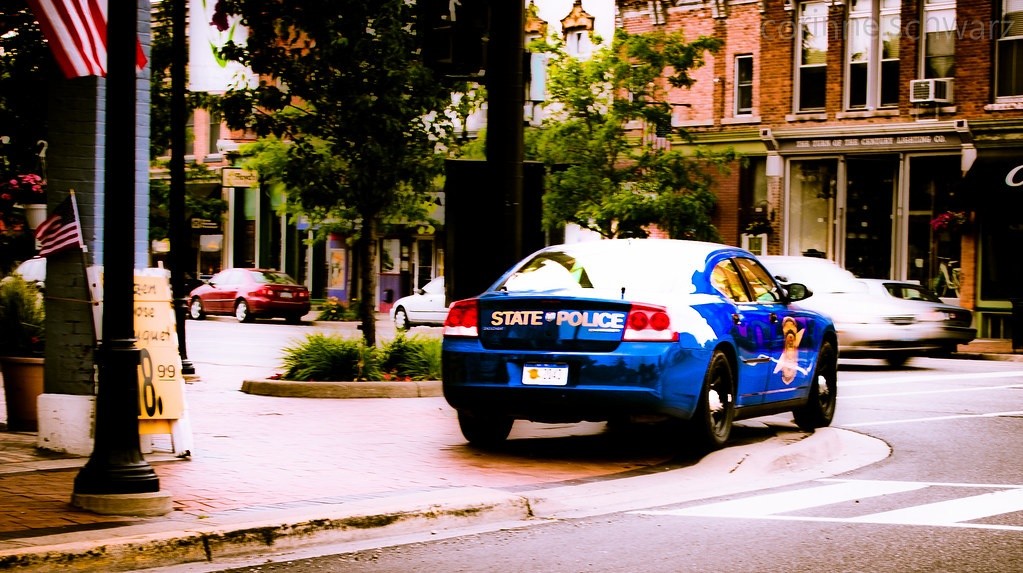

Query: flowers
0;173;47;204
929;210;967;232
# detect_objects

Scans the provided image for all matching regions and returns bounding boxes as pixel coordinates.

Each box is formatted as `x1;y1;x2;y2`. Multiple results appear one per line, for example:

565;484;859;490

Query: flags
29;0;147;79
34;195;82;256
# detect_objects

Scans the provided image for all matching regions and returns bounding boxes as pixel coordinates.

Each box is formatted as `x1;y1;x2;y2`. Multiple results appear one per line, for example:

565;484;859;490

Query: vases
23;204;47;230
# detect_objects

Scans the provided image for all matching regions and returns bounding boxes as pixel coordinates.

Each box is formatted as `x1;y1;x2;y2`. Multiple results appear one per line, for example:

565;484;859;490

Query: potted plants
0;269;45;432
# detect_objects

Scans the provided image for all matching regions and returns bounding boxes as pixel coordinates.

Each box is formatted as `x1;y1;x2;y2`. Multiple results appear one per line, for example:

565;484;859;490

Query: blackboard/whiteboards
88;267;187;424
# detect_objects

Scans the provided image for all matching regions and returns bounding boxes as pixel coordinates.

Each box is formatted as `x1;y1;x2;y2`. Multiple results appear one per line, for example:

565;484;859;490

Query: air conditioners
910;78;953;104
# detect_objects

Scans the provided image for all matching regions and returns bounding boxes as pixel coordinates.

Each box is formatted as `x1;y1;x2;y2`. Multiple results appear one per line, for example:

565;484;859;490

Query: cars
390;274;449;332
441;239;839;450
173;266;311;325
754;254;977;368
